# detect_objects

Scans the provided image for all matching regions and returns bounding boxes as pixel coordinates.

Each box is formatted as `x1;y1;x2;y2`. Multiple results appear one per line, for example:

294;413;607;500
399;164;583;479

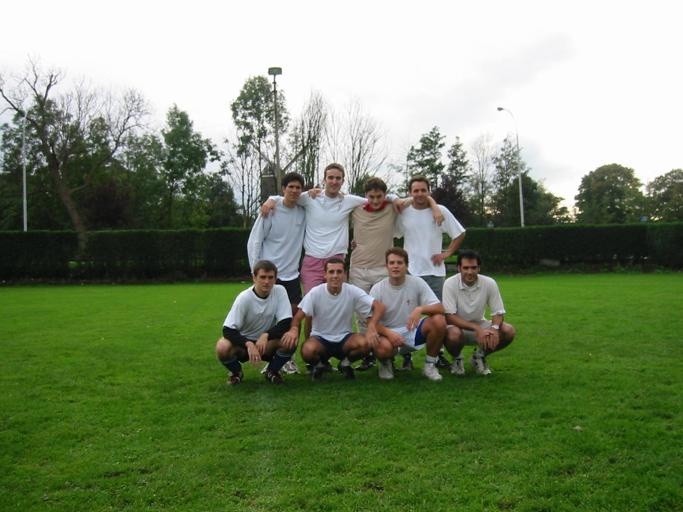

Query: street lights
268;67;287;195
497;108;524;227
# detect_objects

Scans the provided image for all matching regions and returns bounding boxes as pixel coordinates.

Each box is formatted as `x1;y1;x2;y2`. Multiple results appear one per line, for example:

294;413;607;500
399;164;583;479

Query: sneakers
282;356;298;375
449;355;464;376
469;347;491;376
226;370;243;385
377;358;394;379
260;365;283;385
422;361;442;381
355;353;376;370
401;352;413;371
434;355;452;372
304;359;356;382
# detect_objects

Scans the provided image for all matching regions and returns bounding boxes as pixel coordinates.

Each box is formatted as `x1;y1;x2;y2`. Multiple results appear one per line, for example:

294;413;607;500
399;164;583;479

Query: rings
255;357;258;360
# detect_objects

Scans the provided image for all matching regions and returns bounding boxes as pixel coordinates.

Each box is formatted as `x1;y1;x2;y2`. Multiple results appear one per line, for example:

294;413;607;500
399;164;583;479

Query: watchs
490;324;501;330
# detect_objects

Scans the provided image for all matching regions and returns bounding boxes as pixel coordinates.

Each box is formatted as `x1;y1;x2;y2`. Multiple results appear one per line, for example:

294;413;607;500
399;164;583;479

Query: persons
215;260;296;385
442;252;516;378
308;176;447;372
260;162;406;375
278;259;386;382
350;178;466;372
245;170;319;377
363;247;447;382
